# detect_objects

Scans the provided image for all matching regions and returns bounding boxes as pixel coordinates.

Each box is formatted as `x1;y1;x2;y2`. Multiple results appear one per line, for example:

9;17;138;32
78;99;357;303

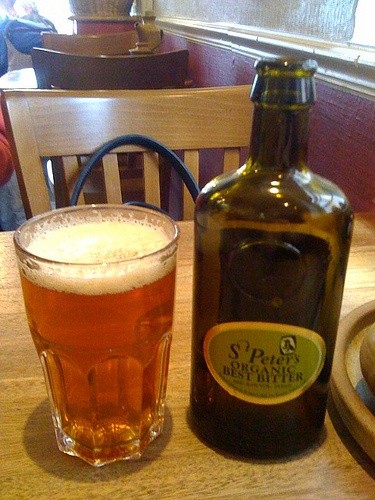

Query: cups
13;204;180;466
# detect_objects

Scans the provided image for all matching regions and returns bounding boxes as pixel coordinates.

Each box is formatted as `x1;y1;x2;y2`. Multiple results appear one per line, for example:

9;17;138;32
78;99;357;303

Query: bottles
190;57;355;459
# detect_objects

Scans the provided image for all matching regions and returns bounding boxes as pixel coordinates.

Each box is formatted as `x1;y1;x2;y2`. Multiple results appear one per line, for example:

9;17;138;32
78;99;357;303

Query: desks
0;212;375;500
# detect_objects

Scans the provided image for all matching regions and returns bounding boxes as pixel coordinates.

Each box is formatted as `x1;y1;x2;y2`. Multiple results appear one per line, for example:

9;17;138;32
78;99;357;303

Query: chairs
31;47;189;215
40;30;137;55
0;83;253;223
